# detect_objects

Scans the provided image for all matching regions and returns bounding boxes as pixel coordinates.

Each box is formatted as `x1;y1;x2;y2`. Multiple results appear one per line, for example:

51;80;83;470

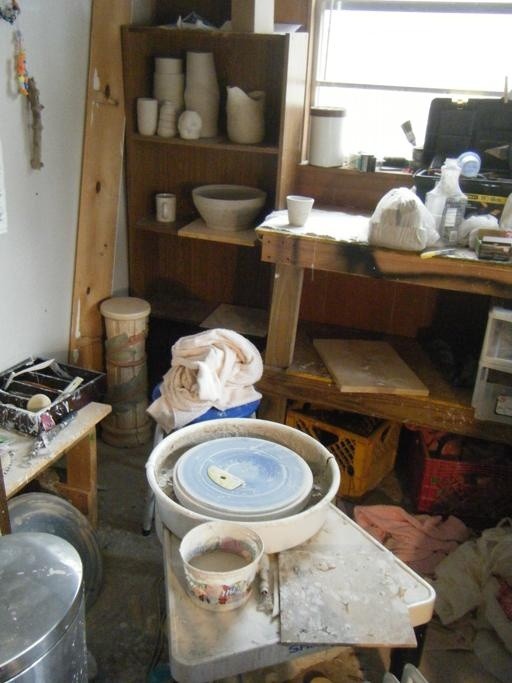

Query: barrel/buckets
177;520;265;612
425;150;483;247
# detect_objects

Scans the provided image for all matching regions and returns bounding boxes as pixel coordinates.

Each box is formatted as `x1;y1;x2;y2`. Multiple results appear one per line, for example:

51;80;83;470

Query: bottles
425;158;469;245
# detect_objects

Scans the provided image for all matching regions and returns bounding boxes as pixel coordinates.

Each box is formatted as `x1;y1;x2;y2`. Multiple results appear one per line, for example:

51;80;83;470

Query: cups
136;98;158;137
156;193;177;223
286;195;314;225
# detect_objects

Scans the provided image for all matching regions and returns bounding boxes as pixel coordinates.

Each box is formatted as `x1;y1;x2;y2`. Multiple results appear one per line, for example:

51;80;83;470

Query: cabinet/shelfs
255;207;512;443
119;28;308;340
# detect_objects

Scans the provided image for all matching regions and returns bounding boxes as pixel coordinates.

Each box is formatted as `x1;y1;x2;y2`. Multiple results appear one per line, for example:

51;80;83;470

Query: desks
159;523;440;682
0;398;112;534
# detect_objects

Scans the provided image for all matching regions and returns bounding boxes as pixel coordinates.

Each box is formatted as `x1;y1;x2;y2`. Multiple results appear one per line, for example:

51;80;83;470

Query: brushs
1;358;55;391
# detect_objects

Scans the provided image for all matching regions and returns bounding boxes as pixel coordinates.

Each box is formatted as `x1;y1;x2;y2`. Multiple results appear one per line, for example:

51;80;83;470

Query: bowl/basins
192;185;266;232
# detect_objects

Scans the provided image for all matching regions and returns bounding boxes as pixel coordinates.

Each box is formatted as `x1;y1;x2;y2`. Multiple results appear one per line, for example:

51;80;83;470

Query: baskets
284;401;403;500
409;431;512;519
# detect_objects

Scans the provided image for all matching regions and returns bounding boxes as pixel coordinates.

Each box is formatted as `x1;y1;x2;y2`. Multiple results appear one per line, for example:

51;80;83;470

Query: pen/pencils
420;249;456;259
